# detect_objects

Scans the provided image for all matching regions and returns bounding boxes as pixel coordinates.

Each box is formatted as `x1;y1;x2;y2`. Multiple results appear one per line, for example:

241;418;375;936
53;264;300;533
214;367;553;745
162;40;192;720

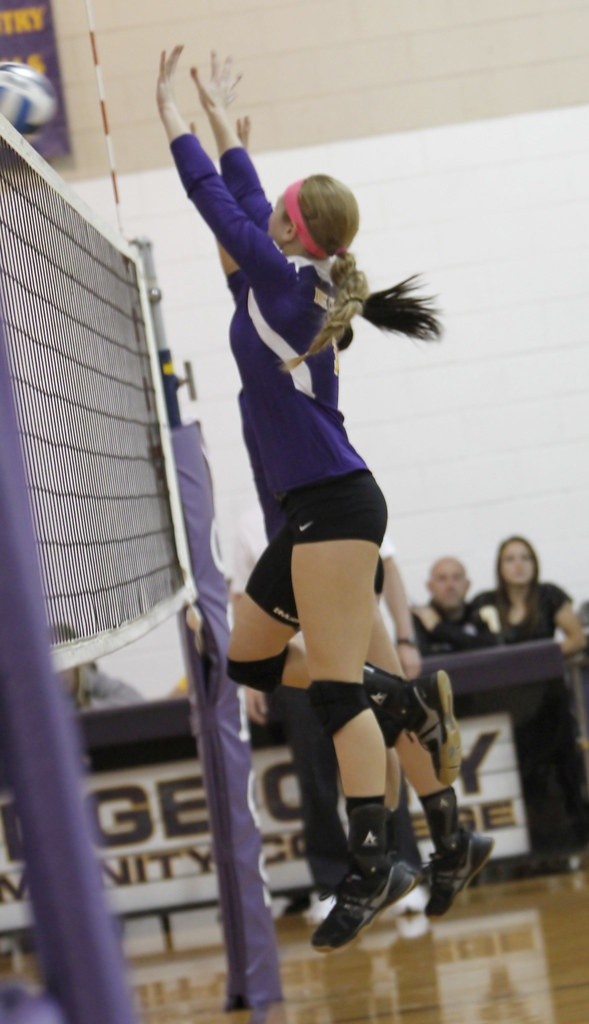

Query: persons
414;558;495;655
470;537;586;658
157;42;499;956
49;622;145;710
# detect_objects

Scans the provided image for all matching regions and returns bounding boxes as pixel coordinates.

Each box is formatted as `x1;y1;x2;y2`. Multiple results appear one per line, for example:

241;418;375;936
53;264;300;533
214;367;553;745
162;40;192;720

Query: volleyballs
0;61;59;144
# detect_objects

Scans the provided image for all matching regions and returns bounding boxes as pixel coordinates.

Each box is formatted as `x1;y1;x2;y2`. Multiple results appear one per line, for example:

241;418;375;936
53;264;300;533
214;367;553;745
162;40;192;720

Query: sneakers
311;859;420;951
405;670;462;786
425;833;494;920
